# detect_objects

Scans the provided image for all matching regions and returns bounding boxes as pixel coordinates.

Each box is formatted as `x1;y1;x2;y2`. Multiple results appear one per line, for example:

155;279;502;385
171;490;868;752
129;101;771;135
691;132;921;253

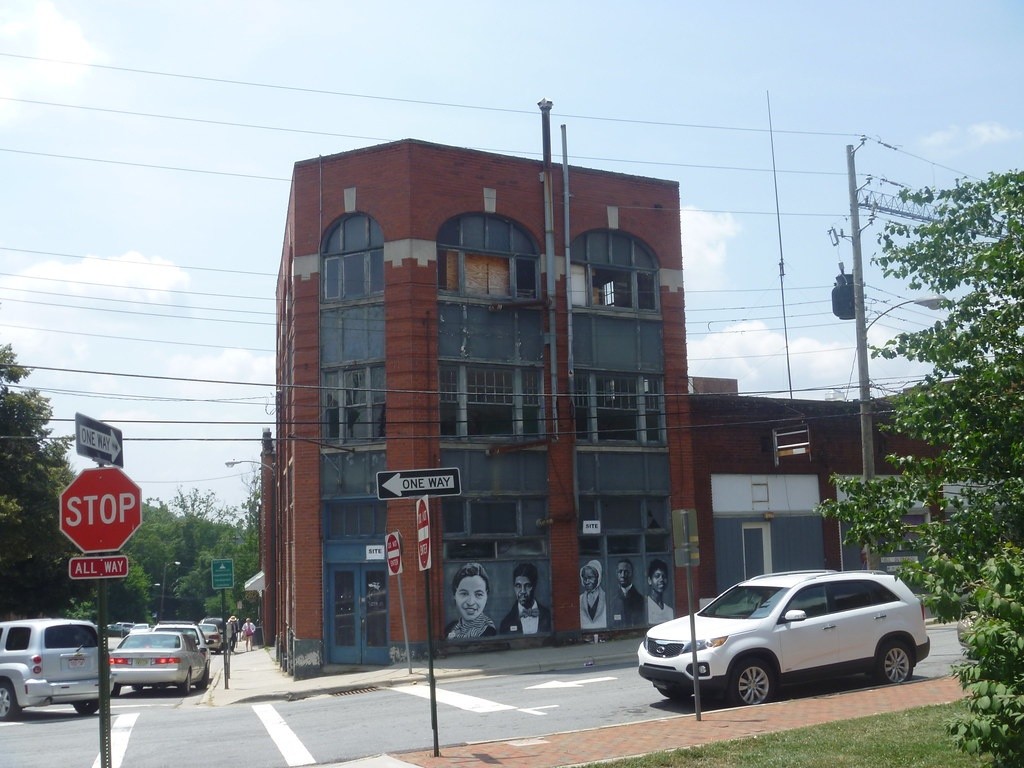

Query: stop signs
415;494;432;570
57;466;142;553
383;531;402;576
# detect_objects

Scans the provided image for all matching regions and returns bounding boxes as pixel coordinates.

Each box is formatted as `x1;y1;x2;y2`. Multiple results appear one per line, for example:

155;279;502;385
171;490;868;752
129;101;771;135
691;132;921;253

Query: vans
0;619;115;722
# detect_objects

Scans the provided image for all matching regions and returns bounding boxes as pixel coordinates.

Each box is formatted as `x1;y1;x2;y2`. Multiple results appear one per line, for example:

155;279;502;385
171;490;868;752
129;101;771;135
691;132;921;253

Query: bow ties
522;609;540;618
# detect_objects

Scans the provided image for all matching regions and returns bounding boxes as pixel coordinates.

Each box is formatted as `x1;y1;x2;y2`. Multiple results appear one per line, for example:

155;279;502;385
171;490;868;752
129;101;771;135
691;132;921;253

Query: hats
579;560;602;591
230;616;237;621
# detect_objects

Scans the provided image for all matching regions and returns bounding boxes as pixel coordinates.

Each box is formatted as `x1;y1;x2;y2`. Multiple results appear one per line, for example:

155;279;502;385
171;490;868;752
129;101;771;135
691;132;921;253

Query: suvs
637;570;932;708
200;617;229;650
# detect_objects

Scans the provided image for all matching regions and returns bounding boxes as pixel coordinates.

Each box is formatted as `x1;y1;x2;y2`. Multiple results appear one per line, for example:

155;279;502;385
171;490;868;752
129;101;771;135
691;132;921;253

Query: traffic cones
854;294;948;568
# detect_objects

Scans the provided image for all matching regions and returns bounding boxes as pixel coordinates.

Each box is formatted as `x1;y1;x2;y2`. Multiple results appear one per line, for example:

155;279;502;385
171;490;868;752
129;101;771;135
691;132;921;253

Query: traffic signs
376;467;463;500
73;410;124;469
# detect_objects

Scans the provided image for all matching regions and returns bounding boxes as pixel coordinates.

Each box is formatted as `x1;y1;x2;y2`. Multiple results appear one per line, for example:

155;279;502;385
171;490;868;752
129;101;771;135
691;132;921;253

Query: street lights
160;561;181;622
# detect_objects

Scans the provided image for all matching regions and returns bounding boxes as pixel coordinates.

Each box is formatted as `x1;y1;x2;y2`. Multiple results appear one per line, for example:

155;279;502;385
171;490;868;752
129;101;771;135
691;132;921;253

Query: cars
198;624;224;654
156;621;197;626
109;632;208;698
108;622;154;650
153;624;215;672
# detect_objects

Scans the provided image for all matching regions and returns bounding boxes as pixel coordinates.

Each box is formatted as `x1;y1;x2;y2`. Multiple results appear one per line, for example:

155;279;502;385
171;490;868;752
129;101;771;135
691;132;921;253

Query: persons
446;563;497;638
580;559;673;628
500;561;550;634
242;618;255;651
226;616;240;652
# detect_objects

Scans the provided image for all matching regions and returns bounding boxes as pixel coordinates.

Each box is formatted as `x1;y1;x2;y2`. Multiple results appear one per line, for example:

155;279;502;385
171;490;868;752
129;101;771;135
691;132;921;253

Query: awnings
244;570;265;590
